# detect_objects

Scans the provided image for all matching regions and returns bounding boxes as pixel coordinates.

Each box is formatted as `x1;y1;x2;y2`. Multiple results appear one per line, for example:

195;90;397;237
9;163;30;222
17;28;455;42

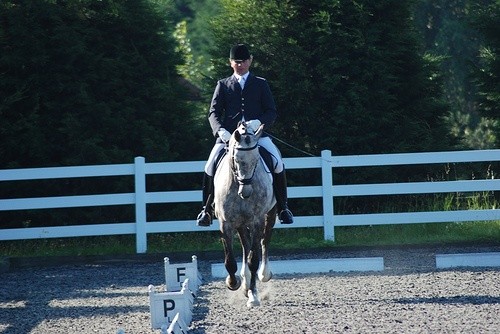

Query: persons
197;44;294;227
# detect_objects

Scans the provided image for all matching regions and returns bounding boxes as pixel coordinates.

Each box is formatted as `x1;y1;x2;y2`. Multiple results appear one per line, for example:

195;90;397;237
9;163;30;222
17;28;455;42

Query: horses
214;120;277;305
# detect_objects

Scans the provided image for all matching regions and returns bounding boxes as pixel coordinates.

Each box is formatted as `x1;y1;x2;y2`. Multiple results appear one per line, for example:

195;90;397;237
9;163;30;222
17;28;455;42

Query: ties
238;76;245;90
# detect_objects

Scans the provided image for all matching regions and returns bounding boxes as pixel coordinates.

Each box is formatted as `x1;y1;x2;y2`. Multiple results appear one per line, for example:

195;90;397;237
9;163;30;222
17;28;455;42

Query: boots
198;172;213;225
273;164;292;222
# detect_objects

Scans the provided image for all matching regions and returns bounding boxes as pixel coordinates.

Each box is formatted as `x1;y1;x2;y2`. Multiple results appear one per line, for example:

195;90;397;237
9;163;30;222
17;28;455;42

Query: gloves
246;118;262;132
218;128;231;142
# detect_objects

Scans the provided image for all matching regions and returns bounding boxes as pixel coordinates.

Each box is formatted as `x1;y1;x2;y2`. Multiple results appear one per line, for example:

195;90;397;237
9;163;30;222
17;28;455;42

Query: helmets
229;44;249;61
